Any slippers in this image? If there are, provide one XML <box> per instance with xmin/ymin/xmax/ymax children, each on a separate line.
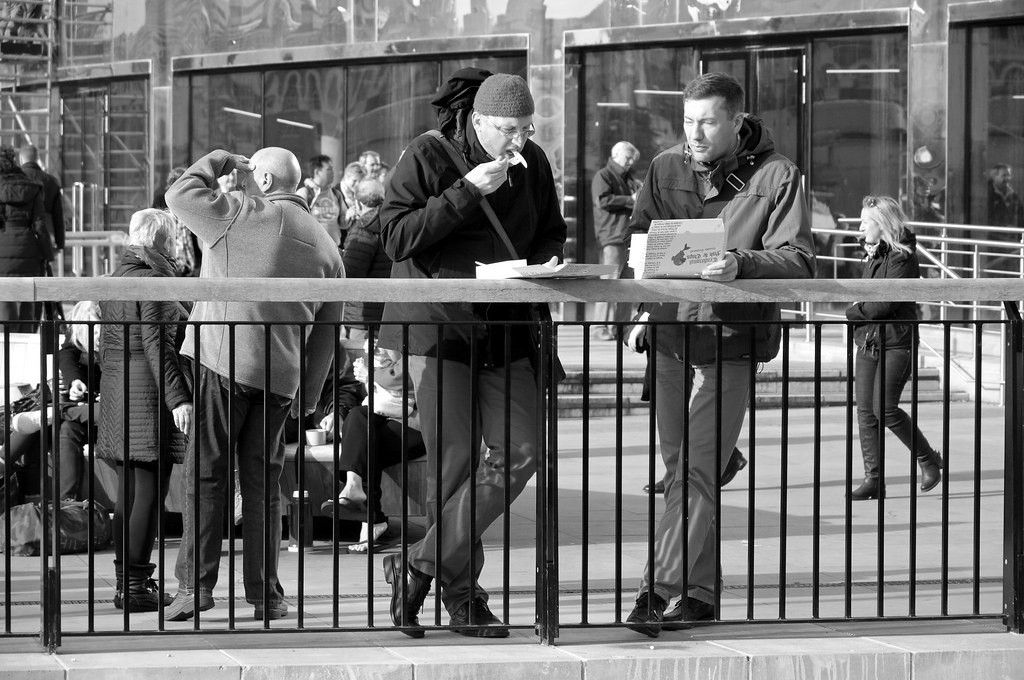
<box><xmin>347</xmin><ymin>532</ymin><xmax>400</xmax><ymax>555</ymax></box>
<box><xmin>320</xmin><ymin>497</ymin><xmax>366</xmax><ymax>521</ymax></box>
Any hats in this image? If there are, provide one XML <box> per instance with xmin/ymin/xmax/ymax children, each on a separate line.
<box><xmin>474</xmin><ymin>73</ymin><xmax>535</xmax><ymax>118</ymax></box>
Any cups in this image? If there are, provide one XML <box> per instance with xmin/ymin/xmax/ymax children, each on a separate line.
<box><xmin>306</xmin><ymin>428</ymin><xmax>326</xmax><ymax>446</ymax></box>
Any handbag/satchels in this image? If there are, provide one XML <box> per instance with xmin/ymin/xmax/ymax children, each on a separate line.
<box><xmin>0</xmin><ymin>498</ymin><xmax>114</xmax><ymax>559</ymax></box>
<box><xmin>44</xmin><ymin>263</ymin><xmax>68</xmax><ymax>335</ymax></box>
<box><xmin>533</xmin><ymin>318</ymin><xmax>566</xmax><ymax>387</ymax></box>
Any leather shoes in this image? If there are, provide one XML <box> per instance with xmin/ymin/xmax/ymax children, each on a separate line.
<box><xmin>450</xmin><ymin>598</ymin><xmax>510</xmax><ymax>637</ymax></box>
<box><xmin>384</xmin><ymin>553</ymin><xmax>430</xmax><ymax>638</ymax></box>
<box><xmin>721</xmin><ymin>448</ymin><xmax>747</xmax><ymax>485</ymax></box>
<box><xmin>644</xmin><ymin>479</ymin><xmax>665</xmax><ymax>494</ymax></box>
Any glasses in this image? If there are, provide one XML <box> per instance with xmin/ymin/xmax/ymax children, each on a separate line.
<box><xmin>484</xmin><ymin>115</ymin><xmax>536</xmax><ymax>141</ymax></box>
<box><xmin>867</xmin><ymin>199</ymin><xmax>879</xmax><ymax>209</ymax></box>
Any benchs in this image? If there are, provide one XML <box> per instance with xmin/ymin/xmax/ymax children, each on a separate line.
<box><xmin>76</xmin><ymin>441</ymin><xmax>431</xmax><ymax>519</ymax></box>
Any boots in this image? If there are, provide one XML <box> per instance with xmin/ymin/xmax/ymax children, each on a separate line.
<box><xmin>122</xmin><ymin>565</ymin><xmax>176</xmax><ymax>612</ymax></box>
<box><xmin>114</xmin><ymin>558</ymin><xmax>123</xmax><ymax>609</ymax></box>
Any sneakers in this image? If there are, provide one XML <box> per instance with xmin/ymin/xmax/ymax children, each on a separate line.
<box><xmin>625</xmin><ymin>591</ymin><xmax>668</xmax><ymax>638</ymax></box>
<box><xmin>254</xmin><ymin>601</ymin><xmax>288</xmax><ymax>620</ymax></box>
<box><xmin>164</xmin><ymin>586</ymin><xmax>215</xmax><ymax>620</ymax></box>
<box><xmin>661</xmin><ymin>597</ymin><xmax>716</xmax><ymax>630</ymax></box>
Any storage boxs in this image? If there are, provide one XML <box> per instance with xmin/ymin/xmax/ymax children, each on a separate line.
<box><xmin>468</xmin><ymin>258</ymin><xmax>616</xmax><ymax>277</ymax></box>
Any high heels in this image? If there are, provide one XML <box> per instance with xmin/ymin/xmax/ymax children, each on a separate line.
<box><xmin>918</xmin><ymin>451</ymin><xmax>944</xmax><ymax>492</ymax></box>
<box><xmin>852</xmin><ymin>477</ymin><xmax>887</xmax><ymax>500</ymax></box>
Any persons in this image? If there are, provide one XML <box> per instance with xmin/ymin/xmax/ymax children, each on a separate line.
<box><xmin>96</xmin><ymin>209</ymin><xmax>193</xmax><ymax>612</ymax></box>
<box><xmin>845</xmin><ymin>196</ymin><xmax>942</xmax><ymax>499</ymax></box>
<box><xmin>591</xmin><ymin>141</ymin><xmax>640</xmax><ymax>340</ymax></box>
<box><xmin>0</xmin><ymin>145</ymin><xmax>65</xmax><ymax>334</ymax></box>
<box><xmin>0</xmin><ymin>299</ymin><xmax>426</xmax><ymax>552</ymax></box>
<box><xmin>988</xmin><ymin>163</ymin><xmax>1023</xmax><ymax>315</ymax></box>
<box><xmin>165</xmin><ymin>147</ymin><xmax>347</xmax><ymax>620</ymax></box>
<box><xmin>623</xmin><ymin>73</ymin><xmax>815</xmax><ymax>631</ymax></box>
<box><xmin>154</xmin><ymin>151</ymin><xmax>394</xmax><ymax>341</ymax></box>
<box><xmin>378</xmin><ymin>66</ymin><xmax>567</xmax><ymax>638</ymax></box>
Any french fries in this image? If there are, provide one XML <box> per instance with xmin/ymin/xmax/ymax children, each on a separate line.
<box><xmin>511</xmin><ymin>150</ymin><xmax>527</xmax><ymax>168</ymax></box>
